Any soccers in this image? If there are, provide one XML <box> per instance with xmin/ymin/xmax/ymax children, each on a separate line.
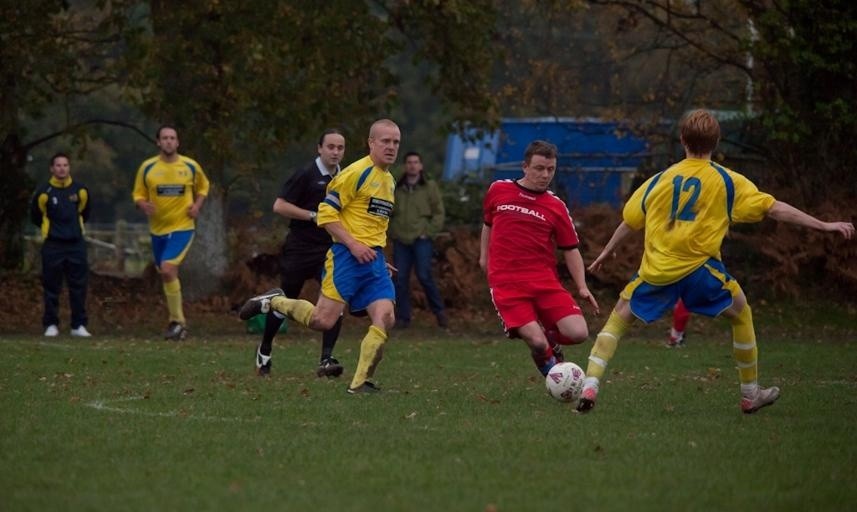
<box><xmin>546</xmin><ymin>362</ymin><xmax>586</xmax><ymax>403</ymax></box>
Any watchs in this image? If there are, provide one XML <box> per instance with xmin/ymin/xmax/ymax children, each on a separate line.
<box><xmin>310</xmin><ymin>211</ymin><xmax>316</xmax><ymax>222</ymax></box>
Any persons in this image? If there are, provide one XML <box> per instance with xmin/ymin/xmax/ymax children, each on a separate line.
<box><xmin>132</xmin><ymin>124</ymin><xmax>211</xmax><ymax>341</ymax></box>
<box><xmin>388</xmin><ymin>151</ymin><xmax>452</xmax><ymax>330</ymax></box>
<box><xmin>254</xmin><ymin>127</ymin><xmax>345</xmax><ymax>377</ymax></box>
<box><xmin>478</xmin><ymin>140</ymin><xmax>601</xmax><ymax>379</ymax></box>
<box><xmin>239</xmin><ymin>119</ymin><xmax>401</xmax><ymax>396</ymax></box>
<box><xmin>29</xmin><ymin>152</ymin><xmax>93</xmax><ymax>338</ymax></box>
<box><xmin>668</xmin><ymin>299</ymin><xmax>689</xmax><ymax>345</ymax></box>
<box><xmin>575</xmin><ymin>107</ymin><xmax>855</xmax><ymax>415</ymax></box>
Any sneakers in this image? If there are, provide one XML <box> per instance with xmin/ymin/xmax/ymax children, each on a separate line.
<box><xmin>346</xmin><ymin>380</ymin><xmax>381</xmax><ymax>395</ymax></box>
<box><xmin>44</xmin><ymin>325</ymin><xmax>59</xmax><ymax>338</ymax></box>
<box><xmin>70</xmin><ymin>325</ymin><xmax>91</xmax><ymax>338</ymax></box>
<box><xmin>317</xmin><ymin>356</ymin><xmax>342</xmax><ymax>378</ymax></box>
<box><xmin>542</xmin><ymin>326</ymin><xmax>564</xmax><ymax>362</ymax></box>
<box><xmin>255</xmin><ymin>342</ymin><xmax>272</xmax><ymax>377</ymax></box>
<box><xmin>164</xmin><ymin>321</ymin><xmax>188</xmax><ymax>341</ymax></box>
<box><xmin>576</xmin><ymin>381</ymin><xmax>599</xmax><ymax>413</ymax></box>
<box><xmin>665</xmin><ymin>332</ymin><xmax>687</xmax><ymax>348</ymax></box>
<box><xmin>239</xmin><ymin>288</ymin><xmax>286</xmax><ymax>321</ymax></box>
<box><xmin>740</xmin><ymin>385</ymin><xmax>780</xmax><ymax>414</ymax></box>
